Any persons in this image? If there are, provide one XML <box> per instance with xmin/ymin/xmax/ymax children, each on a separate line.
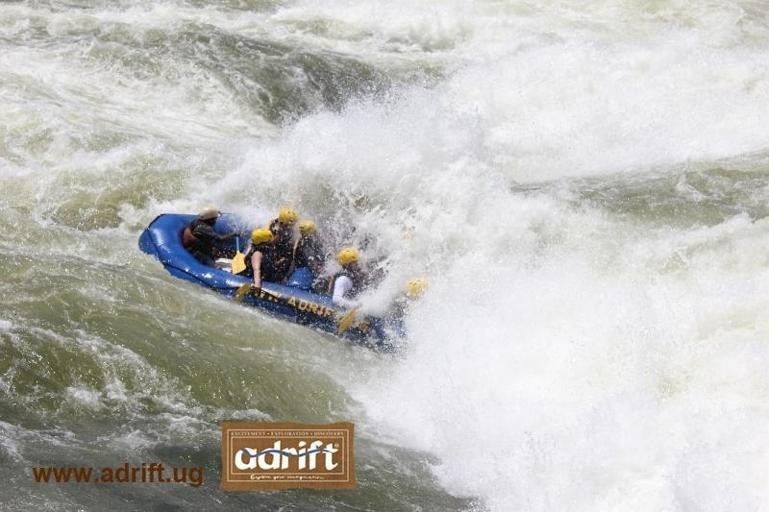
<box><xmin>183</xmin><ymin>203</ymin><xmax>427</xmax><ymax>339</ymax></box>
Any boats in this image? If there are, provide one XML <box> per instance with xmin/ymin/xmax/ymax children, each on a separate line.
<box><xmin>139</xmin><ymin>212</ymin><xmax>407</xmax><ymax>353</ymax></box>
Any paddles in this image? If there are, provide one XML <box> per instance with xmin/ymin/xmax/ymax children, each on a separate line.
<box><xmin>235</xmin><ymin>256</ymin><xmax>288</xmax><ymax>303</ymax></box>
<box><xmin>339</xmin><ymin>303</ymin><xmax>362</xmax><ymax>335</ymax></box>
<box><xmin>231</xmin><ymin>218</ymin><xmax>247</xmax><ymax>275</ymax></box>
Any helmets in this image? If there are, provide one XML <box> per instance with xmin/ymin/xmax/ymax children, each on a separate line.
<box><xmin>337</xmin><ymin>247</ymin><xmax>359</xmax><ymax>266</ymax></box>
<box><xmin>251</xmin><ymin>228</ymin><xmax>274</xmax><ymax>245</ymax></box>
<box><xmin>298</xmin><ymin>220</ymin><xmax>317</xmax><ymax>235</ymax></box>
<box><xmin>199</xmin><ymin>207</ymin><xmax>219</xmax><ymax>220</ymax></box>
<box><xmin>403</xmin><ymin>278</ymin><xmax>430</xmax><ymax>298</ymax></box>
<box><xmin>278</xmin><ymin>207</ymin><xmax>299</xmax><ymax>226</ymax></box>
